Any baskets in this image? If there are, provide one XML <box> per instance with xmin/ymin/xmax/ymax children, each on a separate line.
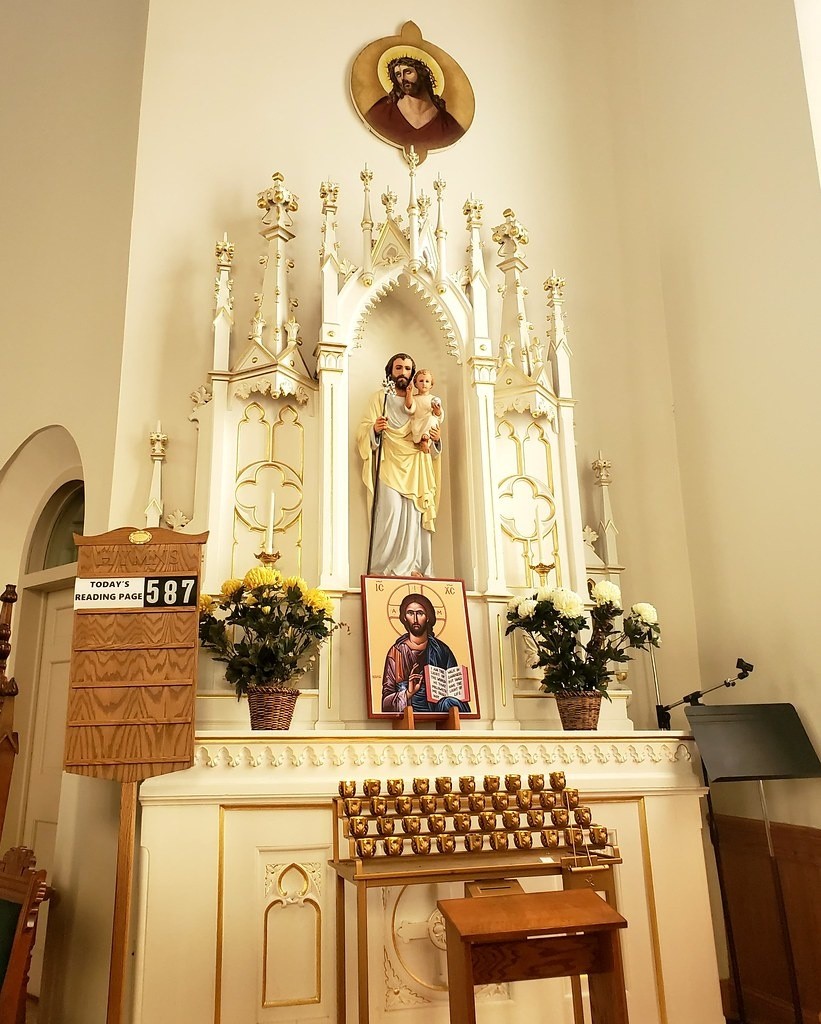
<box><xmin>554</xmin><ymin>691</ymin><xmax>603</xmax><ymax>731</ymax></box>
<box><xmin>247</xmin><ymin>686</ymin><xmax>301</xmax><ymax>730</ymax></box>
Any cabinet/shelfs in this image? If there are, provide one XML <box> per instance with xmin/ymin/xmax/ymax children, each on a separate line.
<box><xmin>328</xmin><ymin>790</ymin><xmax>629</xmax><ymax>1024</ymax></box>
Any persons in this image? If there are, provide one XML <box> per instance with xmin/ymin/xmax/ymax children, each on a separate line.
<box><xmin>404</xmin><ymin>369</ymin><xmax>445</xmax><ymax>455</ymax></box>
<box><xmin>357</xmin><ymin>352</ymin><xmax>442</xmax><ymax>578</ymax></box>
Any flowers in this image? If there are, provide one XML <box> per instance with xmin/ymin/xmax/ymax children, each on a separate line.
<box><xmin>199</xmin><ymin>566</ymin><xmax>350</xmax><ymax>703</ymax></box>
<box><xmin>503</xmin><ymin>579</ymin><xmax>662</xmax><ymax>703</ymax></box>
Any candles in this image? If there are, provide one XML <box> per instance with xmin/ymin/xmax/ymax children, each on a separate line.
<box><xmin>536</xmin><ymin>503</ymin><xmax>545</xmax><ymax>565</ymax></box>
<box><xmin>265</xmin><ymin>491</ymin><xmax>274</xmax><ymax>555</ymax></box>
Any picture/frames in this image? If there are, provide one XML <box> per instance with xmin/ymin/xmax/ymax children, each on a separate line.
<box><xmin>361</xmin><ymin>574</ymin><xmax>481</xmax><ymax>719</ymax></box>
